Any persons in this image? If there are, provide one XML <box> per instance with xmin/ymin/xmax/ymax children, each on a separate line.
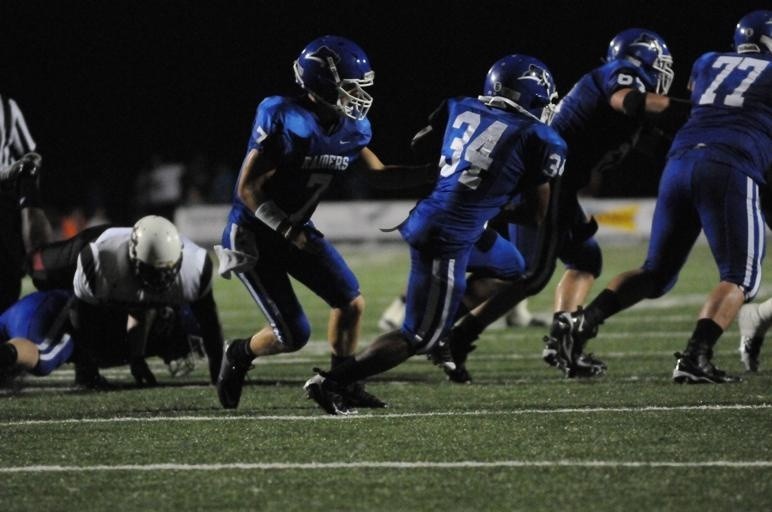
<box><xmin>738</xmin><ymin>293</ymin><xmax>772</xmax><ymax>372</ymax></box>
<box><xmin>550</xmin><ymin>10</ymin><xmax>771</xmax><ymax>386</ymax></box>
<box><xmin>1</xmin><ymin>85</ymin><xmax>42</xmax><ymax>313</ymax></box>
<box><xmin>378</xmin><ymin>195</ymin><xmax>552</xmax><ymax>335</ymax></box>
<box><xmin>215</xmin><ymin>34</ymin><xmax>439</xmax><ymax>410</ymax></box>
<box><xmin>303</xmin><ymin>54</ymin><xmax>569</xmax><ymax>418</ymax></box>
<box><xmin>427</xmin><ymin>27</ymin><xmax>695</xmax><ymax>386</ymax></box>
<box><xmin>2</xmin><ymin>289</ymin><xmax>216</xmax><ymax>376</ymax></box>
<box><xmin>0</xmin><ymin>152</ymin><xmax>224</xmax><ymax>391</ymax></box>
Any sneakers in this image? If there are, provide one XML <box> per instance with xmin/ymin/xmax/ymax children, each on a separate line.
<box><xmin>323</xmin><ymin>356</ymin><xmax>388</xmax><ymax>410</ymax></box>
<box><xmin>302</xmin><ymin>374</ymin><xmax>351</xmax><ymax>416</ymax></box>
<box><xmin>738</xmin><ymin>303</ymin><xmax>768</xmax><ymax>373</ymax></box>
<box><xmin>66</xmin><ymin>371</ymin><xmax>107</xmax><ymax>394</ymax></box>
<box><xmin>216</xmin><ymin>337</ymin><xmax>253</xmax><ymax>409</ymax></box>
<box><xmin>1</xmin><ymin>344</ymin><xmax>20</xmax><ymax>388</ymax></box>
<box><xmin>445</xmin><ymin>340</ymin><xmax>473</xmax><ymax>383</ymax></box>
<box><xmin>129</xmin><ymin>356</ymin><xmax>156</xmax><ymax>387</ymax></box>
<box><xmin>377</xmin><ymin>297</ymin><xmax>407</xmax><ymax>334</ymax></box>
<box><xmin>673</xmin><ymin>356</ymin><xmax>744</xmax><ymax>385</ymax></box>
<box><xmin>508</xmin><ymin>313</ymin><xmax>549</xmax><ymax>330</ymax></box>
<box><xmin>551</xmin><ymin>309</ymin><xmax>596</xmax><ymax>367</ymax></box>
<box><xmin>428</xmin><ymin>332</ymin><xmax>456</xmax><ymax>371</ymax></box>
<box><xmin>540</xmin><ymin>326</ymin><xmax>608</xmax><ymax>378</ymax></box>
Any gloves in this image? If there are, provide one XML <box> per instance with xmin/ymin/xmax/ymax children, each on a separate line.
<box><xmin>258</xmin><ymin>203</ymin><xmax>323</xmax><ymax>249</ymax></box>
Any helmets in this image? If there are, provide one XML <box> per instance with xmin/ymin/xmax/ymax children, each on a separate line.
<box><xmin>477</xmin><ymin>52</ymin><xmax>558</xmax><ymax>126</ymax></box>
<box><xmin>293</xmin><ymin>33</ymin><xmax>377</xmax><ymax>121</ymax></box>
<box><xmin>733</xmin><ymin>10</ymin><xmax>772</xmax><ymax>60</ymax></box>
<box><xmin>605</xmin><ymin>28</ymin><xmax>674</xmax><ymax>97</ymax></box>
<box><xmin>130</xmin><ymin>215</ymin><xmax>185</xmax><ymax>291</ymax></box>
